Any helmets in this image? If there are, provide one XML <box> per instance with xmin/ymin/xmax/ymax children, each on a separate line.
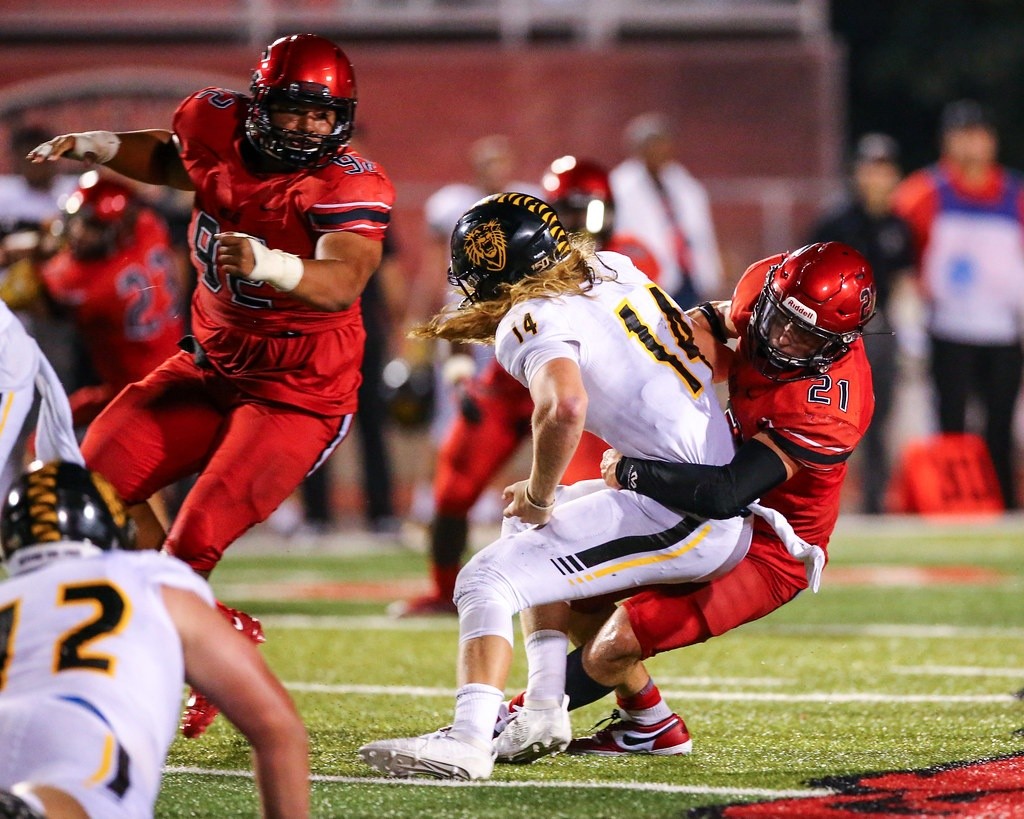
<box><xmin>451</xmin><ymin>191</ymin><xmax>571</xmax><ymax>300</ymax></box>
<box><xmin>543</xmin><ymin>154</ymin><xmax>613</xmax><ymax>209</ymax></box>
<box><xmin>66</xmin><ymin>178</ymin><xmax>137</xmax><ymax>261</ymax></box>
<box><xmin>1</xmin><ymin>458</ymin><xmax>127</xmax><ymax>560</ymax></box>
<box><xmin>245</xmin><ymin>31</ymin><xmax>357</xmax><ymax>171</ymax></box>
<box><xmin>747</xmin><ymin>241</ymin><xmax>878</xmax><ymax>384</ymax></box>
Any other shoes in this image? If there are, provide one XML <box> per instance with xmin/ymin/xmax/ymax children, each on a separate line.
<box><xmin>388</xmin><ymin>594</ymin><xmax>457</xmax><ymax>619</ymax></box>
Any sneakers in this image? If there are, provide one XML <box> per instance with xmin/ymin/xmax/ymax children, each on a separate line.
<box><xmin>434</xmin><ymin>698</ymin><xmax>514</xmax><ymax>740</ymax></box>
<box><xmin>492</xmin><ymin>695</ymin><xmax>571</xmax><ymax>764</ymax></box>
<box><xmin>564</xmin><ymin>708</ymin><xmax>693</xmax><ymax>756</ymax></box>
<box><xmin>357</xmin><ymin>726</ymin><xmax>499</xmax><ymax>779</ymax></box>
<box><xmin>180</xmin><ymin>608</ymin><xmax>266</xmax><ymax>739</ymax></box>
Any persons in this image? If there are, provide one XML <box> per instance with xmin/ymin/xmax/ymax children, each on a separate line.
<box><xmin>358</xmin><ymin>190</ymin><xmax>759</xmax><ymax>781</ymax></box>
<box><xmin>0</xmin><ymin>30</ymin><xmax>397</xmax><ymax>740</ymax></box>
<box><xmin>398</xmin><ymin>110</ymin><xmax>727</xmax><ymax>554</ymax></box>
<box><xmin>387</xmin><ymin>152</ymin><xmax>663</xmax><ymax>618</ymax></box>
<box><xmin>439</xmin><ymin>241</ymin><xmax>874</xmax><ymax>741</ymax></box>
<box><xmin>798</xmin><ymin>98</ymin><xmax>1024</xmax><ymax>514</ymax></box>
<box><xmin>0</xmin><ymin>298</ymin><xmax>89</xmax><ymax>567</ymax></box>
<box><xmin>0</xmin><ymin>463</ymin><xmax>310</xmax><ymax>819</ymax></box>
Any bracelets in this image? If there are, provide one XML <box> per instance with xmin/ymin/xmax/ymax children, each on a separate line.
<box><xmin>524</xmin><ymin>484</ymin><xmax>557</xmax><ymax>512</ymax></box>
